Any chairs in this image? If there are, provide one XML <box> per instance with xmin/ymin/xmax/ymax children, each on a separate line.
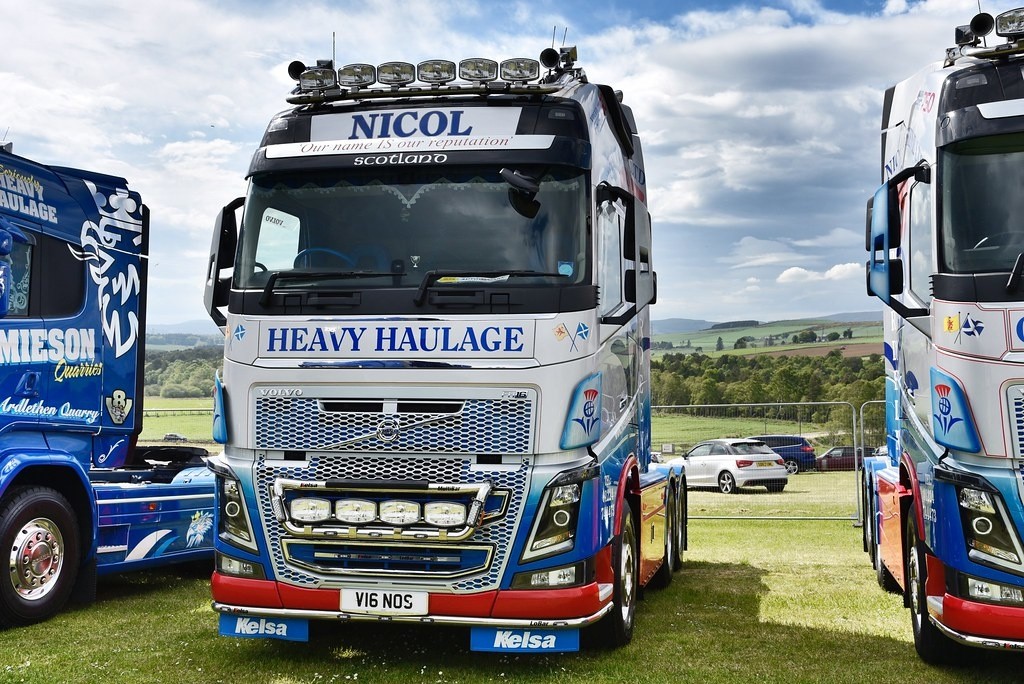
<box><xmin>338</xmin><ymin>240</ymin><xmax>396</xmax><ymax>275</ymax></box>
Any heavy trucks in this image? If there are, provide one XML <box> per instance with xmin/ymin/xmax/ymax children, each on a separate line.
<box><xmin>198</xmin><ymin>36</ymin><xmax>692</xmax><ymax>661</ymax></box>
<box><xmin>852</xmin><ymin>1</ymin><xmax>1024</xmax><ymax>670</ymax></box>
<box><xmin>0</xmin><ymin>143</ymin><xmax>220</xmax><ymax>626</ymax></box>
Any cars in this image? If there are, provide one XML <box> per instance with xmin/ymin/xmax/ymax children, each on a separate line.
<box><xmin>665</xmin><ymin>436</ymin><xmax>790</xmax><ymax>494</ymax></box>
<box><xmin>814</xmin><ymin>444</ymin><xmax>868</xmax><ymax>472</ymax></box>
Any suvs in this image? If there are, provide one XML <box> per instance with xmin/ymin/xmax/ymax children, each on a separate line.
<box><xmin>736</xmin><ymin>433</ymin><xmax>818</xmax><ymax>476</ymax></box>
